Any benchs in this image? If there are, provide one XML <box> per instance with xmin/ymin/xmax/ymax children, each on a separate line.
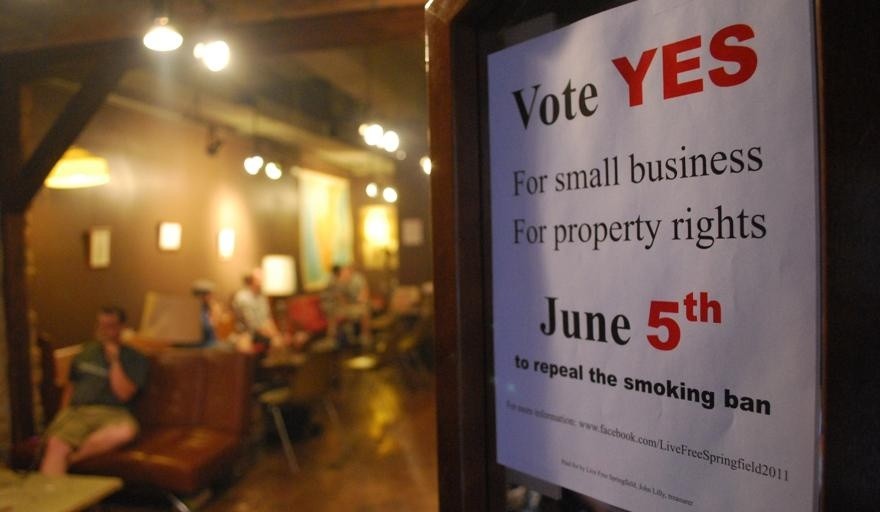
<box><xmin>7</xmin><ymin>331</ymin><xmax>257</xmax><ymax>500</ymax></box>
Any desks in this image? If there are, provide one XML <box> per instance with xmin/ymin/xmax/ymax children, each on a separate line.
<box><xmin>0</xmin><ymin>468</ymin><xmax>125</xmax><ymax>511</ymax></box>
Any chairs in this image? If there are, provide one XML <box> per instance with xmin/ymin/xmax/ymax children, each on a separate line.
<box><xmin>256</xmin><ymin>332</ymin><xmax>391</xmax><ymax>475</ymax></box>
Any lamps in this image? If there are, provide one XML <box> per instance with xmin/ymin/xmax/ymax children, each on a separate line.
<box><xmin>42</xmin><ymin>142</ymin><xmax>112</xmax><ymax>191</ymax></box>
<box><xmin>142</xmin><ymin>0</ymin><xmax>184</xmax><ymax>52</ymax></box>
